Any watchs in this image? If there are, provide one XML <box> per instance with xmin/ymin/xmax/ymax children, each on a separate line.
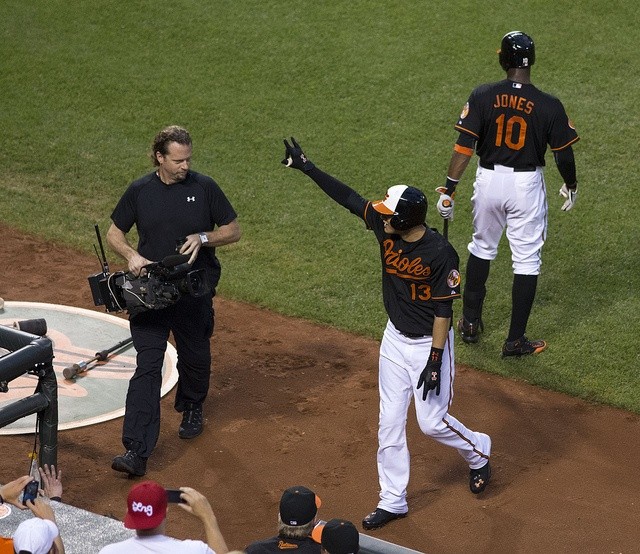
<box><xmin>199</xmin><ymin>232</ymin><xmax>209</xmax><ymax>245</ymax></box>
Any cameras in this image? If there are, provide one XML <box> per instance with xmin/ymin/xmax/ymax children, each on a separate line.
<box><xmin>88</xmin><ymin>224</ymin><xmax>211</xmax><ymax>314</ymax></box>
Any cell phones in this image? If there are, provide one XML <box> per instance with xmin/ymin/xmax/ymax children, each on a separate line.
<box><xmin>22</xmin><ymin>480</ymin><xmax>39</xmax><ymax>507</ymax></box>
<box><xmin>164</xmin><ymin>488</ymin><xmax>188</xmax><ymax>503</ymax></box>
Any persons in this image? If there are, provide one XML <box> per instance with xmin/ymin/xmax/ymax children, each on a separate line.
<box><xmin>98</xmin><ymin>479</ymin><xmax>228</xmax><ymax>554</ymax></box>
<box><xmin>281</xmin><ymin>136</ymin><xmax>491</xmax><ymax>529</ymax></box>
<box><xmin>0</xmin><ymin>474</ymin><xmax>65</xmax><ymax>554</ymax></box>
<box><xmin>320</xmin><ymin>519</ymin><xmax>361</xmax><ymax>553</ymax></box>
<box><xmin>36</xmin><ymin>462</ymin><xmax>63</xmax><ymax>502</ymax></box>
<box><xmin>105</xmin><ymin>124</ymin><xmax>243</xmax><ymax>475</ymax></box>
<box><xmin>243</xmin><ymin>484</ymin><xmax>325</xmax><ymax>554</ymax></box>
<box><xmin>436</xmin><ymin>29</ymin><xmax>581</xmax><ymax>358</ymax></box>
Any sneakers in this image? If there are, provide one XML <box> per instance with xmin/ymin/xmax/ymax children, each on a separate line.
<box><xmin>457</xmin><ymin>319</ymin><xmax>481</xmax><ymax>343</ymax></box>
<box><xmin>362</xmin><ymin>508</ymin><xmax>409</xmax><ymax>530</ymax></box>
<box><xmin>111</xmin><ymin>449</ymin><xmax>147</xmax><ymax>477</ymax></box>
<box><xmin>469</xmin><ymin>460</ymin><xmax>491</xmax><ymax>494</ymax></box>
<box><xmin>179</xmin><ymin>404</ymin><xmax>203</xmax><ymax>439</ymax></box>
<box><xmin>502</xmin><ymin>336</ymin><xmax>547</xmax><ymax>356</ymax></box>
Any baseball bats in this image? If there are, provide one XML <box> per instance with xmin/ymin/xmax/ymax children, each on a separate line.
<box><xmin>442</xmin><ymin>199</ymin><xmax>451</xmax><ymax>238</ymax></box>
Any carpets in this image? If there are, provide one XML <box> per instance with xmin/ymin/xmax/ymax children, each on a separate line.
<box><xmin>0</xmin><ymin>487</ymin><xmax>137</xmax><ymax>554</ymax></box>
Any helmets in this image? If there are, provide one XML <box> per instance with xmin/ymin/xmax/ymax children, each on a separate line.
<box><xmin>498</xmin><ymin>30</ymin><xmax>534</xmax><ymax>66</ymax></box>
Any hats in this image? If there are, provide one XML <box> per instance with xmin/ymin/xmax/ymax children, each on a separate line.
<box><xmin>372</xmin><ymin>184</ymin><xmax>428</xmax><ymax>229</ymax></box>
<box><xmin>124</xmin><ymin>480</ymin><xmax>167</xmax><ymax>531</ymax></box>
<box><xmin>312</xmin><ymin>518</ymin><xmax>359</xmax><ymax>554</ymax></box>
<box><xmin>280</xmin><ymin>486</ymin><xmax>322</xmax><ymax>527</ymax></box>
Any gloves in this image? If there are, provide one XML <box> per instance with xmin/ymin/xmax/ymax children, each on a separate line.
<box><xmin>434</xmin><ymin>186</ymin><xmax>455</xmax><ymax>221</ymax></box>
<box><xmin>281</xmin><ymin>138</ymin><xmax>314</xmax><ymax>175</ymax></box>
<box><xmin>559</xmin><ymin>183</ymin><xmax>578</xmax><ymax>212</ymax></box>
<box><xmin>417</xmin><ymin>346</ymin><xmax>445</xmax><ymax>401</ymax></box>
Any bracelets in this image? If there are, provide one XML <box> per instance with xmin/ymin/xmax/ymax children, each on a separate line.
<box><xmin>445</xmin><ymin>176</ymin><xmax>459</xmax><ymax>196</ymax></box>
<box><xmin>427</xmin><ymin>346</ymin><xmax>444</xmax><ymax>366</ymax></box>
